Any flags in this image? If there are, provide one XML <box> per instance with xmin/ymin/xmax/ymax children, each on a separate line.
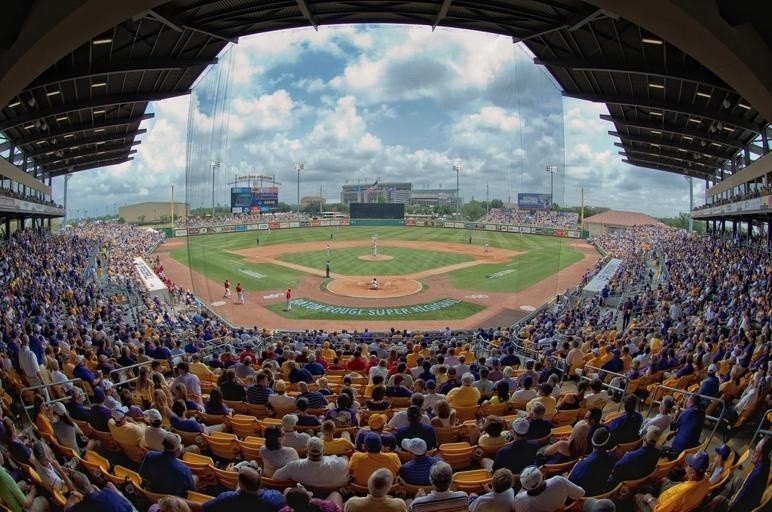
<box><xmin>367</xmin><ymin>182</ymin><xmax>377</xmax><ymax>192</ymax></box>
<box><xmin>388</xmin><ymin>186</ymin><xmax>394</xmax><ymax>191</ymax></box>
<box><xmin>351</xmin><ymin>185</ymin><xmax>359</xmax><ymax>192</ymax></box>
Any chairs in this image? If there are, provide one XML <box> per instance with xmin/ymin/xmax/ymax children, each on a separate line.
<box><xmin>0</xmin><ymin>221</ymin><xmax>772</xmax><ymax>511</ymax></box>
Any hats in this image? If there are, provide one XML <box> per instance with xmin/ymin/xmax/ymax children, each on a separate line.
<box><xmin>307</xmin><ymin>437</ymin><xmax>324</xmax><ymax>455</ymax></box>
<box><xmin>686</xmin><ymin>444</ymin><xmax>732</xmax><ymax>467</ymax></box>
<box><xmin>647</xmin><ymin>425</ymin><xmax>660</xmax><ymax>445</ymax></box>
<box><xmin>520</xmin><ymin>465</ymin><xmax>542</xmax><ymax>490</ymax></box>
<box><xmin>365</xmin><ymin>414</ymin><xmax>388</xmax><ymax>450</ymax></box>
<box><xmin>282</xmin><ymin>414</ymin><xmax>299</xmax><ymax>428</ymax></box>
<box><xmin>708</xmin><ymin>364</ymin><xmax>719</xmax><ymax>373</ymax></box>
<box><xmin>101</xmin><ymin>379</ymin><xmax>114</xmax><ymax>391</ymax></box>
<box><xmin>401</xmin><ymin>438</ymin><xmax>427</xmax><ymax>455</ymax></box>
<box><xmin>592</xmin><ymin>427</ymin><xmax>616</xmax><ymax>450</ymax></box>
<box><xmin>110</xmin><ymin>405</ymin><xmax>162</xmax><ymax>425</ymax></box>
<box><xmin>53</xmin><ymin>402</ymin><xmax>66</xmax><ymax>416</ymax></box>
<box><xmin>163</xmin><ymin>434</ymin><xmax>181</xmax><ymax>451</ymax></box>
<box><xmin>513</xmin><ymin>418</ymin><xmax>529</xmax><ymax>434</ymax></box>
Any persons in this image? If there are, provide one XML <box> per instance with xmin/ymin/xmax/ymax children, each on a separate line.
<box><xmin>0</xmin><ymin>208</ymin><xmax>771</xmax><ymax>510</ymax></box>
